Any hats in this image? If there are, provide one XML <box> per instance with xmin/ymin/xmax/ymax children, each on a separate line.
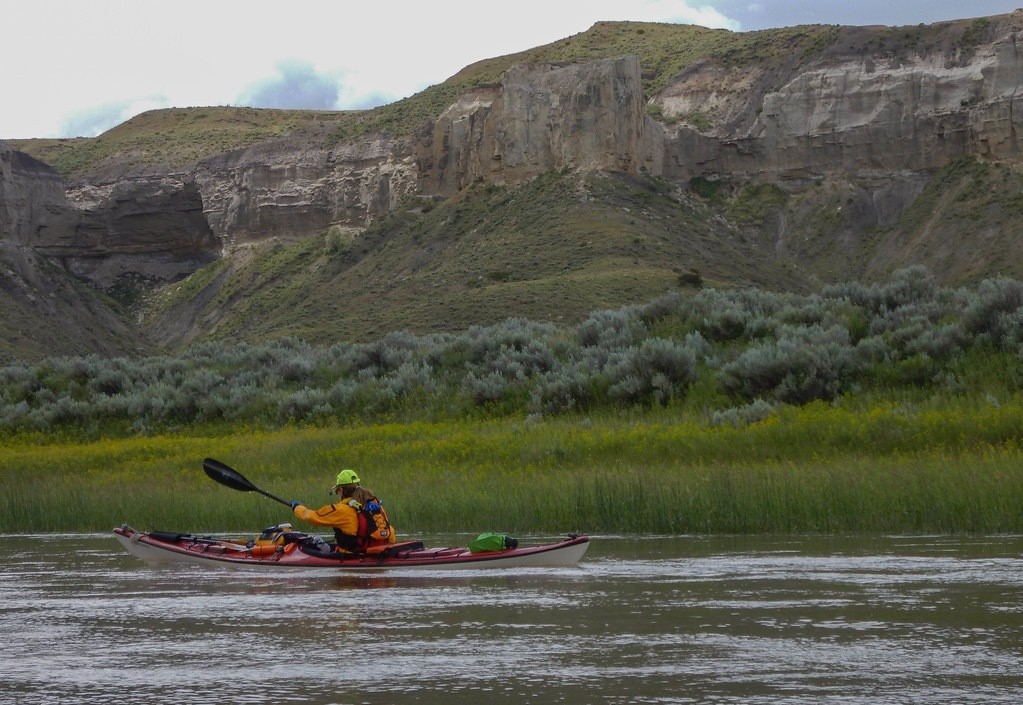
<box><xmin>331</xmin><ymin>470</ymin><xmax>360</xmax><ymax>490</ymax></box>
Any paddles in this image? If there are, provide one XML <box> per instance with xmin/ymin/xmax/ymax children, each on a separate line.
<box><xmin>202</xmin><ymin>456</ymin><xmax>292</xmax><ymax>508</ymax></box>
<box><xmin>140</xmin><ymin>530</ymin><xmax>249</xmax><ymax>551</ymax></box>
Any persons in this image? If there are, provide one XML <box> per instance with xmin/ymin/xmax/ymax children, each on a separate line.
<box><xmin>291</xmin><ymin>469</ymin><xmax>396</xmax><ymax>557</ymax></box>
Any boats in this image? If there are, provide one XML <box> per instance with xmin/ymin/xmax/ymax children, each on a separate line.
<box><xmin>112</xmin><ymin>523</ymin><xmax>591</xmax><ymax>571</ymax></box>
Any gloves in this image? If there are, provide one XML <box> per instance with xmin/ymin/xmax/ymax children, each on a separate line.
<box><xmin>291</xmin><ymin>500</ymin><xmax>300</xmax><ymax>509</ymax></box>
